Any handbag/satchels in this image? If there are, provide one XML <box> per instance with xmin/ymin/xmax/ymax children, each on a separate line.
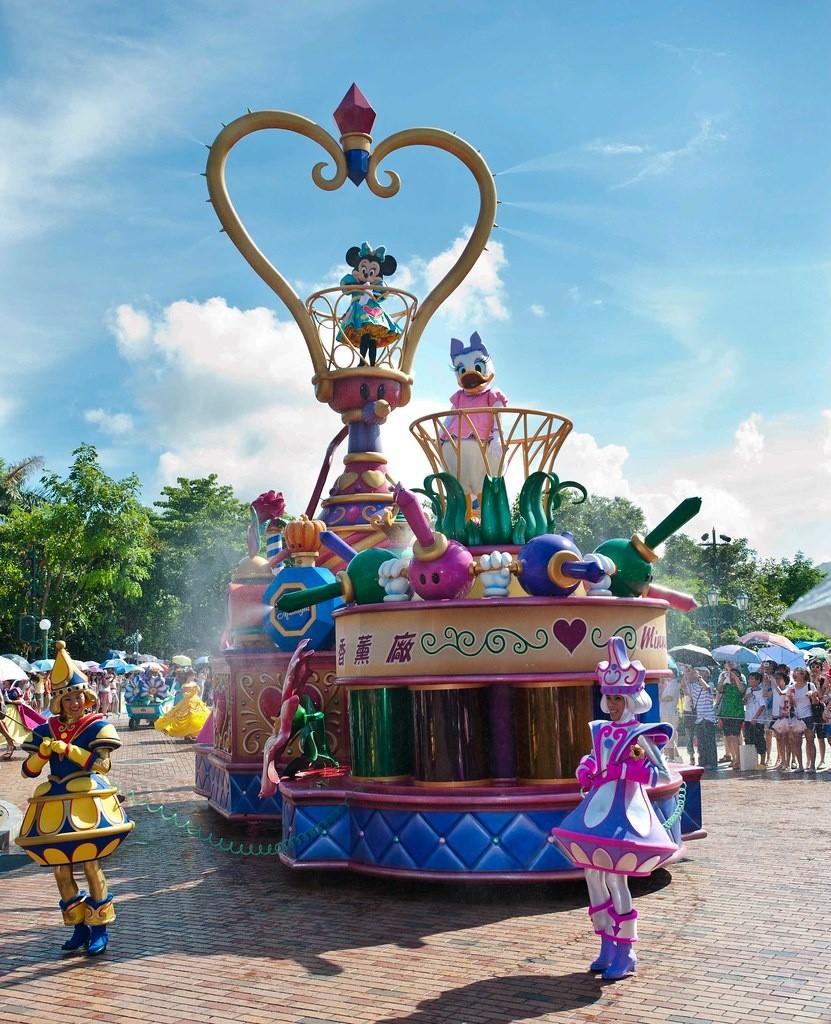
<box><xmin>690</xmin><ymin>709</ymin><xmax>698</xmax><ymax>721</ymax></box>
<box><xmin>812</xmin><ymin>702</ymin><xmax>825</xmax><ymax>723</ymax></box>
<box><xmin>715</xmin><ymin>702</ymin><xmax>723</xmax><ymax>716</ymax></box>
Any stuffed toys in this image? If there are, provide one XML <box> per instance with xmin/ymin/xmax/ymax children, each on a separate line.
<box><xmin>335</xmin><ymin>242</ymin><xmax>397</xmax><ymax>369</ymax></box>
<box><xmin>434</xmin><ymin>331</ymin><xmax>508</xmax><ymax>510</ymax></box>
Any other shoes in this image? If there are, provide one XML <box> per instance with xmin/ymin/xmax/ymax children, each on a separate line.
<box><xmin>733</xmin><ymin>762</ymin><xmax>741</xmax><ymax>770</ymax></box>
<box><xmin>817</xmin><ymin>762</ymin><xmax>828</xmax><ymax>769</ymax></box>
<box><xmin>828</xmin><ymin>768</ymin><xmax>831</xmax><ymax>774</ymax></box>
<box><xmin>704</xmin><ymin>764</ymin><xmax>719</xmax><ymax>771</ymax></box>
<box><xmin>760</xmin><ymin>761</ymin><xmax>817</xmax><ymax>773</ymax></box>
<box><xmin>754</xmin><ymin>763</ymin><xmax>768</xmax><ymax>772</ymax></box>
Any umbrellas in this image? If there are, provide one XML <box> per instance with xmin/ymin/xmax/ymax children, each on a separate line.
<box><xmin>772</xmin><ymin>707</ymin><xmax>807</xmax><ymax>734</ymax></box>
<box><xmin>668</xmin><ymin>630</ymin><xmax>831</xmax><ymax>671</ymax></box>
<box><xmin>0</xmin><ymin>650</ymin><xmax>210</xmax><ymax>681</ymax></box>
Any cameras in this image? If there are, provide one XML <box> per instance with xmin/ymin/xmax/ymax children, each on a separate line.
<box><xmin>724</xmin><ymin>673</ymin><xmax>731</xmax><ymax>677</ymax></box>
<box><xmin>764</xmin><ymin>662</ymin><xmax>769</xmax><ymax>666</ymax></box>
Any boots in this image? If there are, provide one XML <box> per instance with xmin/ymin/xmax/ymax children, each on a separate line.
<box><xmin>589</xmin><ymin>897</ymin><xmax>620</xmax><ymax>972</ymax></box>
<box><xmin>601</xmin><ymin>905</ymin><xmax>641</xmax><ymax>981</ymax></box>
<box><xmin>84</xmin><ymin>892</ymin><xmax>116</xmax><ymax>955</ymax></box>
<box><xmin>57</xmin><ymin>890</ymin><xmax>98</xmax><ymax>952</ymax></box>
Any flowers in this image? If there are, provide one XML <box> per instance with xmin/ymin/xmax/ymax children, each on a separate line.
<box><xmin>124</xmin><ymin>675</ymin><xmax>170</xmax><ymax>705</ymax></box>
<box><xmin>258</xmin><ymin>637</ymin><xmax>339</xmax><ymax>801</ymax></box>
<box><xmin>250</xmin><ymin>490</ymin><xmax>286</xmax><ymax>526</ymax></box>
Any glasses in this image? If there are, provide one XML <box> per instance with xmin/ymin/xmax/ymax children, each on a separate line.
<box><xmin>812</xmin><ymin>665</ymin><xmax>821</xmax><ymax>669</ymax></box>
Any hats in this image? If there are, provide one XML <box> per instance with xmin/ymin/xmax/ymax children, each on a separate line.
<box><xmin>598</xmin><ymin>635</ymin><xmax>647</xmax><ymax>693</ymax></box>
<box><xmin>695</xmin><ymin>666</ymin><xmax>711</xmax><ymax>676</ymax></box>
<box><xmin>46</xmin><ymin>641</ymin><xmax>98</xmax><ymax>713</ymax></box>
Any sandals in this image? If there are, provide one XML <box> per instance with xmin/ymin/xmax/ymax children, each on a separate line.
<box><xmin>718</xmin><ymin>755</ymin><xmax>732</xmax><ymax>764</ymax></box>
<box><xmin>728</xmin><ymin>760</ymin><xmax>736</xmax><ymax>767</ymax></box>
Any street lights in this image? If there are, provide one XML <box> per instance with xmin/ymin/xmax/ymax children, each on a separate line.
<box><xmin>39</xmin><ymin>618</ymin><xmax>52</xmax><ymax>661</ymax></box>
<box><xmin>736</xmin><ymin>589</ymin><xmax>749</xmax><ymax>638</ymax></box>
<box><xmin>134</xmin><ymin>633</ymin><xmax>143</xmax><ymax>665</ymax></box>
<box><xmin>706</xmin><ymin>585</ymin><xmax>719</xmax><ymax>650</ymax></box>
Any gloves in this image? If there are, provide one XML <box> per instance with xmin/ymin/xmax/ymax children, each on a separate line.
<box><xmin>606</xmin><ymin>760</ymin><xmax>651</xmax><ymax>784</ymax></box>
<box><xmin>49</xmin><ymin>739</ymin><xmax>99</xmax><ymax>772</ymax></box>
<box><xmin>577</xmin><ymin>766</ymin><xmax>595</xmax><ymax>788</ymax></box>
<box><xmin>22</xmin><ymin>738</ymin><xmax>54</xmax><ymax>779</ymax></box>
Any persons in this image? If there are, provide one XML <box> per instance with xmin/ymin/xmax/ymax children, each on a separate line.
<box><xmin>0</xmin><ymin>664</ymin><xmax>213</xmax><ymax>758</ymax></box>
<box><xmin>15</xmin><ymin>643</ymin><xmax>123</xmax><ymax>955</ymax></box>
<box><xmin>551</xmin><ymin>636</ymin><xmax>678</xmax><ymax>983</ymax></box>
<box><xmin>659</xmin><ymin>657</ymin><xmax>831</xmax><ymax>773</ymax></box>
